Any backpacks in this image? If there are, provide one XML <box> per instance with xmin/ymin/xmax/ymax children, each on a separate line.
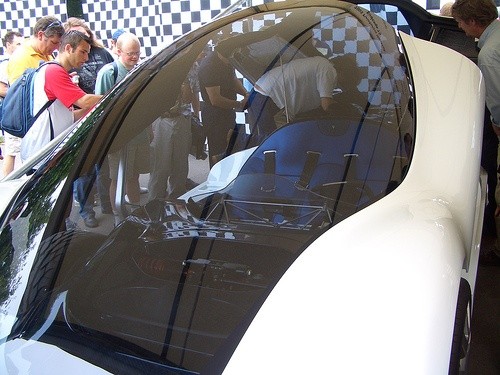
<box><xmin>0</xmin><ymin>59</ymin><xmax>67</xmax><ymax>137</ymax></box>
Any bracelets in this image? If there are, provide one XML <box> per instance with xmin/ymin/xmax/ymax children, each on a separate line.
<box><xmin>243</xmin><ymin>92</ymin><xmax>248</xmax><ymax>96</ymax></box>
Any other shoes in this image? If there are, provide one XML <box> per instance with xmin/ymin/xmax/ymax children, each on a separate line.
<box><xmin>480</xmin><ymin>249</ymin><xmax>500</xmax><ymax>267</ymax></box>
<box><xmin>137</xmin><ymin>184</ymin><xmax>148</xmax><ymax>193</ymax></box>
<box><xmin>74</xmin><ymin>198</ymin><xmax>80</xmax><ymax>206</ymax></box>
<box><xmin>101</xmin><ymin>206</ymin><xmax>114</xmax><ymax>214</ymax></box>
<box><xmin>83</xmin><ymin>213</ymin><xmax>100</xmax><ymax>229</ymax></box>
<box><xmin>186</xmin><ymin>178</ymin><xmax>198</xmax><ymax>190</ymax></box>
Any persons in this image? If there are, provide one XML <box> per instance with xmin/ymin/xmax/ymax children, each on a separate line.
<box><xmin>0</xmin><ymin>17</ymin><xmax>210</xmax><ymax>230</ymax></box>
<box><xmin>198</xmin><ymin>33</ymin><xmax>362</xmax><ymax>170</ymax></box>
<box><xmin>439</xmin><ymin>0</ymin><xmax>500</xmax><ymax>217</ymax></box>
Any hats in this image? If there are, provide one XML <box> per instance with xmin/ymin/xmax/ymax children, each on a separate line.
<box><xmin>112</xmin><ymin>29</ymin><xmax>125</xmax><ymax>40</ymax></box>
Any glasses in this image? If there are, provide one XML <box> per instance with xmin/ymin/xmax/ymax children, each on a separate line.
<box><xmin>119</xmin><ymin>48</ymin><xmax>140</xmax><ymax>55</ymax></box>
<box><xmin>45</xmin><ymin>20</ymin><xmax>62</xmax><ymax>32</ymax></box>
